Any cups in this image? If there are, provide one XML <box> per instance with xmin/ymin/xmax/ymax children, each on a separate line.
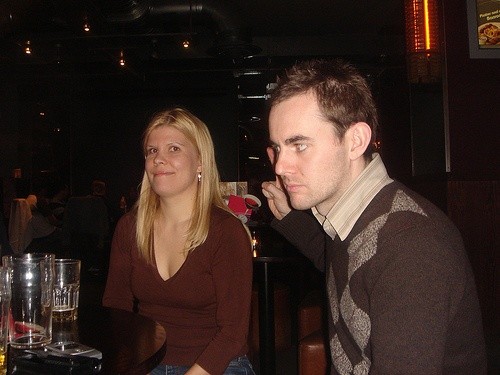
<box><xmin>2</xmin><ymin>252</ymin><xmax>55</xmax><ymax>348</ymax></box>
<box><xmin>0</xmin><ymin>267</ymin><xmax>14</xmax><ymax>375</ymax></box>
<box><xmin>40</xmin><ymin>259</ymin><xmax>81</xmax><ymax>350</ymax></box>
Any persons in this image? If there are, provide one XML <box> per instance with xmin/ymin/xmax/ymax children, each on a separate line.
<box><xmin>102</xmin><ymin>107</ymin><xmax>257</xmax><ymax>375</ymax></box>
<box><xmin>261</xmin><ymin>53</ymin><xmax>488</xmax><ymax>375</ymax></box>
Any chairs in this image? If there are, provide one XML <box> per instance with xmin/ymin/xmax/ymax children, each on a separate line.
<box><xmin>253</xmin><ymin>257</ymin><xmax>279</xmax><ymax>375</ymax></box>
<box><xmin>9</xmin><ymin>198</ymin><xmax>33</xmax><ymax>257</ymax></box>
<box><xmin>62</xmin><ymin>196</ymin><xmax>112</xmax><ymax>283</ymax></box>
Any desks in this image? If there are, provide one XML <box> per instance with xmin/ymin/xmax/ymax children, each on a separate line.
<box><xmin>0</xmin><ymin>300</ymin><xmax>167</xmax><ymax>375</ymax></box>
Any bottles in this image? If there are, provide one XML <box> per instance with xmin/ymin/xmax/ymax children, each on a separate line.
<box><xmin>252</xmin><ymin>232</ymin><xmax>257</xmax><ymax>259</ymax></box>
